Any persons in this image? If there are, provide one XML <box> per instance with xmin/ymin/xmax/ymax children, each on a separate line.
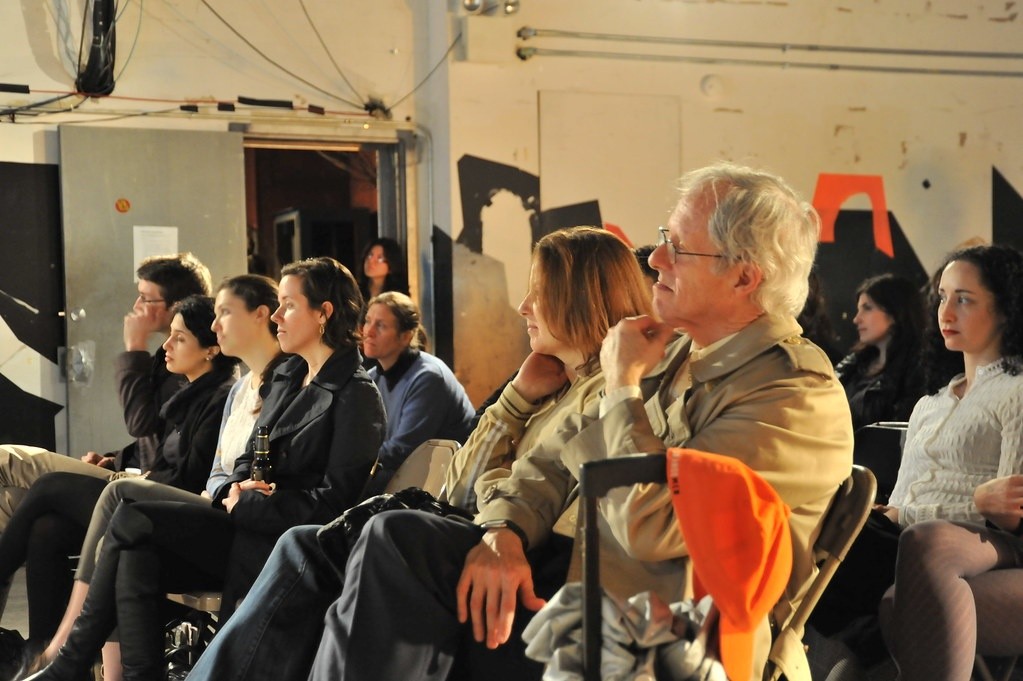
<box><xmin>23</xmin><ymin>259</ymin><xmax>386</xmax><ymax>681</ymax></box>
<box><xmin>184</xmin><ymin>225</ymin><xmax>660</xmax><ymax>681</ymax></box>
<box><xmin>360</xmin><ymin>238</ymin><xmax>410</xmax><ymax>296</ymax></box>
<box><xmin>363</xmin><ymin>292</ymin><xmax>475</xmax><ymax>466</ymax></box>
<box><xmin>0</xmin><ymin>273</ymin><xmax>293</xmax><ymax>681</ymax></box>
<box><xmin>0</xmin><ymin>252</ymin><xmax>241</xmax><ymax>653</ymax></box>
<box><xmin>878</xmin><ymin>475</ymin><xmax>1023</xmax><ymax>681</ymax></box>
<box><xmin>471</xmin><ymin>244</ymin><xmax>658</xmax><ymax>431</ymax></box>
<box><xmin>804</xmin><ymin>245</ymin><xmax>1023</xmax><ymax>681</ymax></box>
<box><xmin>306</xmin><ymin>160</ymin><xmax>853</xmax><ymax>681</ymax></box>
<box><xmin>836</xmin><ymin>274</ymin><xmax>932</xmax><ymax>432</ymax></box>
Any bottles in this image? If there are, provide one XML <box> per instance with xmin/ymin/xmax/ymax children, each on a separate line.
<box><xmin>250</xmin><ymin>426</ymin><xmax>276</xmax><ymax>485</ymax></box>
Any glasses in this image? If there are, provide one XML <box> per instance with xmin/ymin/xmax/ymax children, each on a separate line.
<box><xmin>655</xmin><ymin>226</ymin><xmax>742</xmax><ymax>265</ymax></box>
<box><xmin>366</xmin><ymin>251</ymin><xmax>385</xmax><ymax>264</ymax></box>
<box><xmin>135</xmin><ymin>294</ymin><xmax>165</xmax><ymax>306</ymax></box>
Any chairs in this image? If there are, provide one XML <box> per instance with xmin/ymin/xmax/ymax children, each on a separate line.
<box><xmin>166</xmin><ymin>545</ymin><xmax>257</xmax><ymax>632</ymax></box>
<box><xmin>763</xmin><ymin>466</ymin><xmax>876</xmax><ymax>681</ymax></box>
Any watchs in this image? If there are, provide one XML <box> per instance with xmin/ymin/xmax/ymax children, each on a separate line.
<box><xmin>481</xmin><ymin>520</ymin><xmax>528</xmax><ymax>551</ymax></box>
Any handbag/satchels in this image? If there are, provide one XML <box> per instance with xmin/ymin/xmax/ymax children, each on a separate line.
<box><xmin>165</xmin><ymin>610</ymin><xmax>217</xmax><ymax>681</ymax></box>
<box><xmin>315</xmin><ymin>485</ymin><xmax>475</xmax><ymax>594</ymax></box>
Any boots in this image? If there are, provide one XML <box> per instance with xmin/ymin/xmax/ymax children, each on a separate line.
<box><xmin>113</xmin><ymin>548</ymin><xmax>164</xmax><ymax>681</ymax></box>
<box><xmin>20</xmin><ymin>498</ymin><xmax>156</xmax><ymax>681</ymax></box>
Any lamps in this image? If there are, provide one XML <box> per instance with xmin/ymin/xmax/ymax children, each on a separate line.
<box><xmin>459</xmin><ymin>0</ymin><xmax>520</xmax><ymax>63</ymax></box>
